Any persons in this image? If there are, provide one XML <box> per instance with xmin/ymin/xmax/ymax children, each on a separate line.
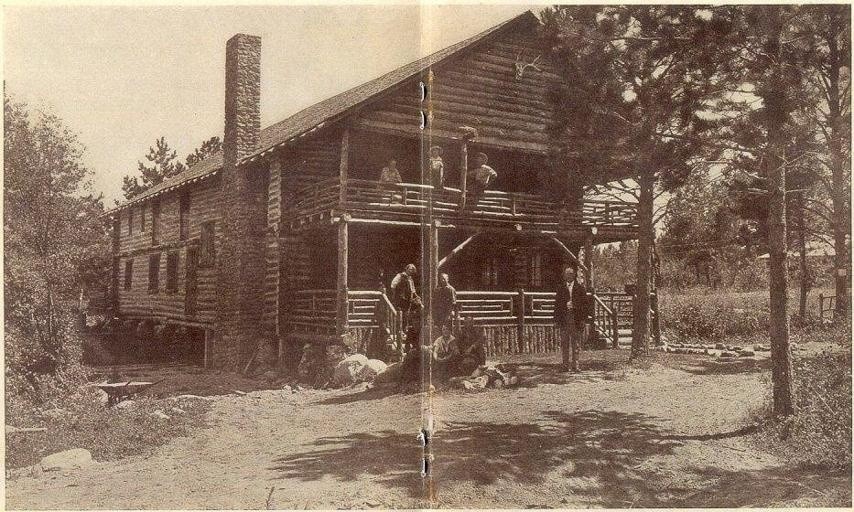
<box><xmin>430</xmin><ymin>144</ymin><xmax>445</xmax><ymax>186</ymax></box>
<box><xmin>466</xmin><ymin>149</ymin><xmax>500</xmax><ymax>190</ymax></box>
<box><xmin>388</xmin><ymin>262</ymin><xmax>423</xmax><ymax>314</ymax></box>
<box><xmin>553</xmin><ymin>267</ymin><xmax>591</xmax><ymax>373</ymax></box>
<box><xmin>401</xmin><ymin>296</ymin><xmax>425</xmax><ymax>352</ymax></box>
<box><xmin>397</xmin><ymin>316</ymin><xmax>433</xmax><ymax>393</ymax></box>
<box><xmin>437</xmin><ymin>272</ymin><xmax>459</xmax><ymax>319</ymax></box>
<box><xmin>459</xmin><ymin>314</ymin><xmax>487</xmax><ymax>366</ymax></box>
<box><xmin>379</xmin><ymin>156</ymin><xmax>407</xmax><ymax>191</ymax></box>
<box><xmin>431</xmin><ymin>323</ymin><xmax>459</xmax><ymax>378</ymax></box>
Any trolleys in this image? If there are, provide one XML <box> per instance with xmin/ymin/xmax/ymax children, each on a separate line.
<box><xmin>95</xmin><ymin>376</ymin><xmax>166</xmax><ymax>404</ymax></box>
